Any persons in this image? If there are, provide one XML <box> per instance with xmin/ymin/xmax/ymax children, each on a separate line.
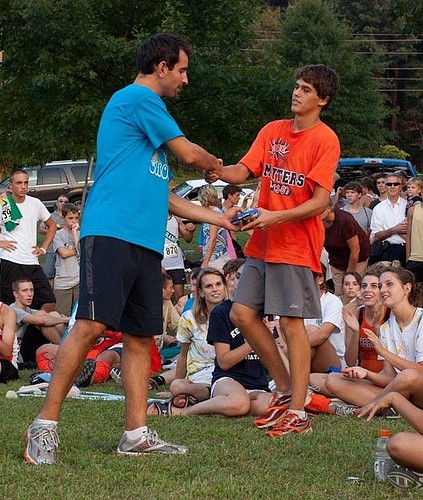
<box><xmin>24</xmin><ymin>32</ymin><xmax>223</xmax><ymax>466</ymax></box>
<box><xmin>355</xmin><ymin>392</ymin><xmax>423</xmax><ymax>490</ymax></box>
<box><xmin>203</xmin><ymin>64</ymin><xmax>341</xmax><ymax>438</ymax></box>
<box><xmin>0</xmin><ymin>169</ymin><xmax>423</xmax><ymax>418</ymax></box>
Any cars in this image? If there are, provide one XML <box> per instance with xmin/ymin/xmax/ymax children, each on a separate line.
<box><xmin>167</xmin><ymin>178</ymin><xmax>255</xmax><ymax>223</ymax></box>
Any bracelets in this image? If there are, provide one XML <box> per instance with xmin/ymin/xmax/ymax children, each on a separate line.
<box><xmin>40</xmin><ymin>247</ymin><xmax>47</xmax><ymax>254</ymax></box>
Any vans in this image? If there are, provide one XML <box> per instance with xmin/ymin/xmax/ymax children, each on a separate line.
<box><xmin>335</xmin><ymin>158</ymin><xmax>418</xmax><ymax>192</ymax></box>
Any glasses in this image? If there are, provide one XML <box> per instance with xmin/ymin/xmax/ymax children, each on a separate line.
<box><xmin>386</xmin><ymin>183</ymin><xmax>401</xmax><ymax>187</ymax></box>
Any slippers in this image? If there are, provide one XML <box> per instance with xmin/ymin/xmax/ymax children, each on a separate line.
<box><xmin>174</xmin><ymin>394</ymin><xmax>199</xmax><ymax>408</ymax></box>
<box><xmin>147</xmin><ymin>400</ymin><xmax>172</xmax><ymax>417</ymax></box>
<box><xmin>149</xmin><ymin>376</ymin><xmax>166</xmax><ymax>389</ymax></box>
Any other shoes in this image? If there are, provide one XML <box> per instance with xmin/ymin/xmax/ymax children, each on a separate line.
<box><xmin>74</xmin><ymin>359</ymin><xmax>95</xmax><ymax>388</ymax></box>
<box><xmin>110</xmin><ymin>367</ymin><xmax>122</xmax><ymax>384</ymax></box>
<box><xmin>386</xmin><ymin>464</ymin><xmax>422</xmax><ymax>491</ymax></box>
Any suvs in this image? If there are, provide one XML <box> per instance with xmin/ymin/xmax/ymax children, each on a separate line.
<box><xmin>1</xmin><ymin>158</ymin><xmax>97</xmax><ymax>216</ymax></box>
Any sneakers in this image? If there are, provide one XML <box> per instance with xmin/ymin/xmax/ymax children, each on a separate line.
<box><xmin>265</xmin><ymin>411</ymin><xmax>313</xmax><ymax>437</ymax></box>
<box><xmin>328</xmin><ymin>398</ymin><xmax>357</xmax><ymax>416</ymax></box>
<box><xmin>117</xmin><ymin>428</ymin><xmax>189</xmax><ymax>455</ymax></box>
<box><xmin>254</xmin><ymin>392</ymin><xmax>291</xmax><ymax>428</ymax></box>
<box><xmin>24</xmin><ymin>418</ymin><xmax>59</xmax><ymax>465</ymax></box>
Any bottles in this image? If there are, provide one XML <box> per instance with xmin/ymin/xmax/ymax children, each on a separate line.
<box><xmin>373</xmin><ymin>430</ymin><xmax>392</xmax><ymax>484</ymax></box>
